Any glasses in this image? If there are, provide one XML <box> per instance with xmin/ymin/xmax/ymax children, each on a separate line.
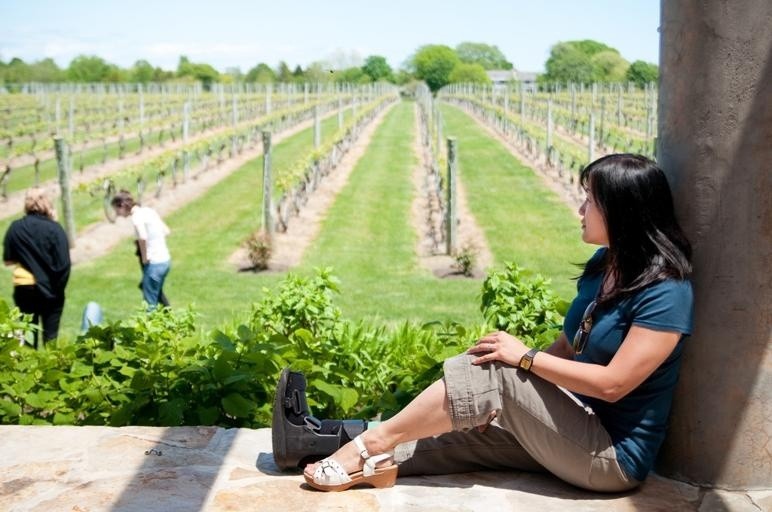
<box><xmin>572</xmin><ymin>299</ymin><xmax>598</xmax><ymax>355</ymax></box>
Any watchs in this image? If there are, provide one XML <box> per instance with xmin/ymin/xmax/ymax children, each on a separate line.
<box><xmin>519</xmin><ymin>348</ymin><xmax>539</xmax><ymax>373</ymax></box>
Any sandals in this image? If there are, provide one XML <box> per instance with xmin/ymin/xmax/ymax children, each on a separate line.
<box><xmin>303</xmin><ymin>434</ymin><xmax>399</xmax><ymax>493</ymax></box>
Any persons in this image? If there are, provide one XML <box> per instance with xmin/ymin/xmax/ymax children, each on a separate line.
<box><xmin>111</xmin><ymin>188</ymin><xmax>172</xmax><ymax>323</ymax></box>
<box><xmin>3</xmin><ymin>187</ymin><xmax>72</xmax><ymax>353</ymax></box>
<box><xmin>271</xmin><ymin>154</ymin><xmax>694</xmax><ymax>492</ymax></box>
